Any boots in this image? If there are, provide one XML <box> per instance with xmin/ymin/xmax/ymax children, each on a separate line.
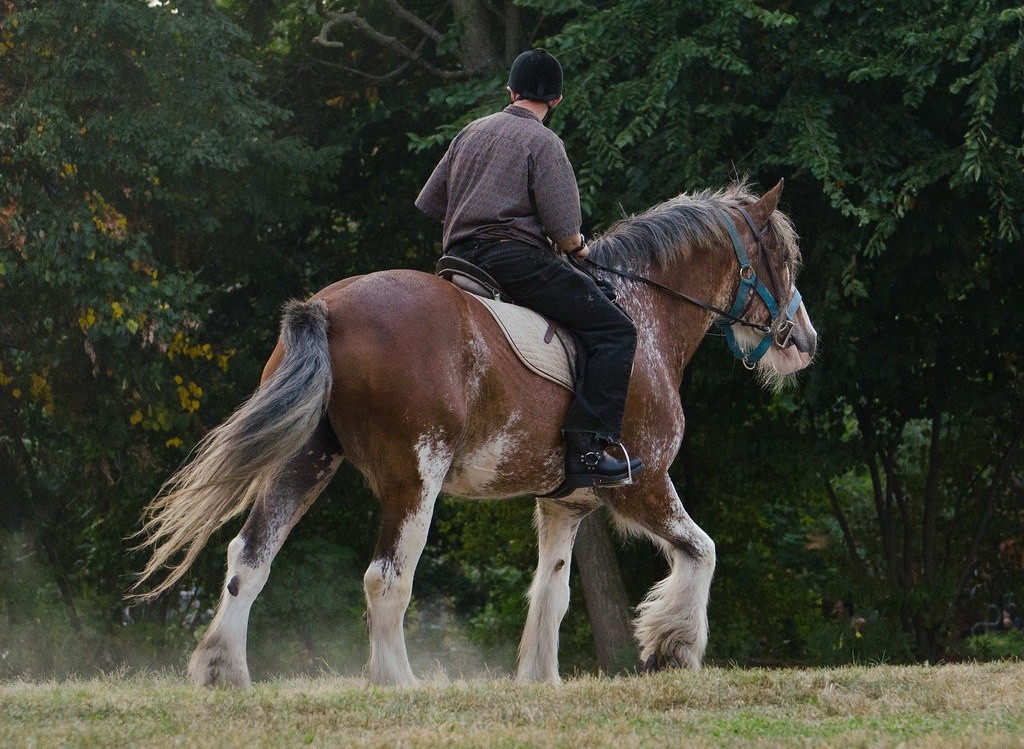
<box><xmin>562</xmin><ymin>429</ymin><xmax>646</xmax><ymax>488</ymax></box>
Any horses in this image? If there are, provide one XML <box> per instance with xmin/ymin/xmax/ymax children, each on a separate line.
<box><xmin>121</xmin><ymin>177</ymin><xmax>818</xmax><ymax>688</ymax></box>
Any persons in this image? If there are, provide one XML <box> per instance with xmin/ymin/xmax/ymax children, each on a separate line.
<box><xmin>414</xmin><ymin>46</ymin><xmax>649</xmax><ymax>486</ymax></box>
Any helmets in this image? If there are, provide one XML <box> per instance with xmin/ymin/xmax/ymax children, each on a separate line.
<box><xmin>508</xmin><ymin>48</ymin><xmax>563</xmax><ymax>100</ymax></box>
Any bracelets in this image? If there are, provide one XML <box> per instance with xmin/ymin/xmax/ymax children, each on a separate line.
<box><xmin>570</xmin><ymin>232</ymin><xmax>586</xmax><ymax>257</ymax></box>
